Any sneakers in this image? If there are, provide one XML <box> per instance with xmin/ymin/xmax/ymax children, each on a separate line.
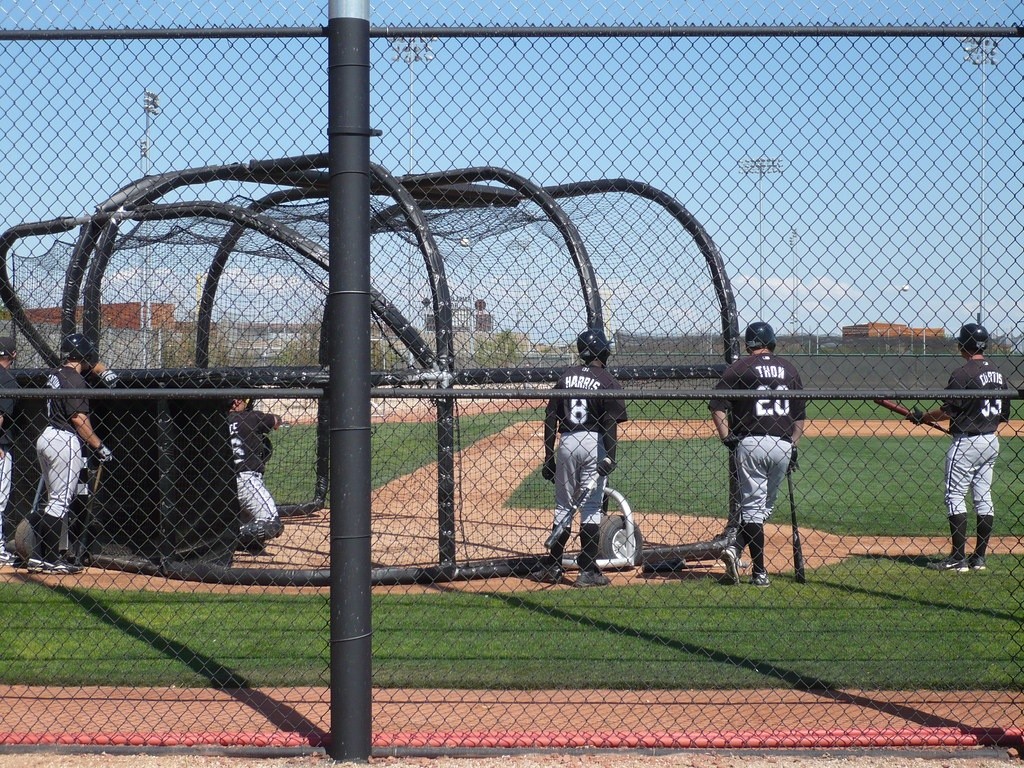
<box><xmin>576</xmin><ymin>570</ymin><xmax>609</xmax><ymax>588</ymax></box>
<box><xmin>927</xmin><ymin>554</ymin><xmax>969</xmax><ymax>572</ymax></box>
<box><xmin>0</xmin><ymin>549</ymin><xmax>18</xmax><ymax>566</ymax></box>
<box><xmin>968</xmin><ymin>553</ymin><xmax>986</xmax><ymax>570</ymax></box>
<box><xmin>42</xmin><ymin>554</ymin><xmax>84</xmax><ymax>574</ymax></box>
<box><xmin>749</xmin><ymin>568</ymin><xmax>771</xmax><ymax>587</ymax></box>
<box><xmin>27</xmin><ymin>558</ymin><xmax>43</xmax><ymax>573</ymax></box>
<box><xmin>721</xmin><ymin>545</ymin><xmax>743</xmax><ymax>584</ymax></box>
<box><xmin>531</xmin><ymin>566</ymin><xmax>564</xmax><ymax>584</ymax></box>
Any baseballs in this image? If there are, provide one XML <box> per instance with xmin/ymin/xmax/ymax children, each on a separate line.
<box><xmin>603</xmin><ymin>457</ymin><xmax>611</xmax><ymax>464</ymax></box>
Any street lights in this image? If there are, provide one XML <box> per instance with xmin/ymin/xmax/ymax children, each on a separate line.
<box><xmin>737</xmin><ymin>157</ymin><xmax>785</xmax><ymax>324</ymax></box>
<box><xmin>959</xmin><ymin>36</ymin><xmax>1000</xmax><ymax>326</ymax></box>
<box><xmin>137</xmin><ymin>88</ymin><xmax>159</xmax><ymax>370</ymax></box>
<box><xmin>390</xmin><ymin>37</ymin><xmax>435</xmax><ymax>372</ymax></box>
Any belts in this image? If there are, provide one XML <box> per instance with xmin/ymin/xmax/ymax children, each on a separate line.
<box><xmin>968</xmin><ymin>431</ymin><xmax>994</xmax><ymax>437</ymax></box>
<box><xmin>243</xmin><ymin>472</ymin><xmax>259</xmax><ymax>477</ymax></box>
<box><xmin>747</xmin><ymin>433</ymin><xmax>792</xmax><ymax>443</ymax></box>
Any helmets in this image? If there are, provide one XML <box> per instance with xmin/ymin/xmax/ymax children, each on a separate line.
<box><xmin>745</xmin><ymin>322</ymin><xmax>776</xmax><ymax>353</ymax></box>
<box><xmin>61</xmin><ymin>333</ymin><xmax>91</xmax><ymax>359</ymax></box>
<box><xmin>577</xmin><ymin>330</ymin><xmax>613</xmax><ymax>361</ymax></box>
<box><xmin>957</xmin><ymin>324</ymin><xmax>988</xmax><ymax>352</ymax></box>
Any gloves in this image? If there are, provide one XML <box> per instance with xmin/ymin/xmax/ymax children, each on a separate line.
<box><xmin>542</xmin><ymin>463</ymin><xmax>557</xmax><ymax>484</ymax></box>
<box><xmin>98</xmin><ymin>444</ymin><xmax>112</xmax><ymax>462</ymax></box>
<box><xmin>723</xmin><ymin>434</ymin><xmax>743</xmax><ymax>454</ymax></box>
<box><xmin>787</xmin><ymin>445</ymin><xmax>799</xmax><ymax>475</ymax></box>
<box><xmin>98</xmin><ymin>369</ymin><xmax>118</xmax><ymax>387</ymax></box>
<box><xmin>596</xmin><ymin>460</ymin><xmax>617</xmax><ymax>476</ymax></box>
<box><xmin>906</xmin><ymin>406</ymin><xmax>925</xmax><ymax>426</ymax></box>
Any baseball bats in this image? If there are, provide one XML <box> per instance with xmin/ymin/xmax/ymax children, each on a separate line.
<box><xmin>873</xmin><ymin>399</ymin><xmax>950</xmax><ymax>435</ymax></box>
<box><xmin>74</xmin><ymin>462</ymin><xmax>104</xmax><ymax>565</ymax></box>
<box><xmin>786</xmin><ymin>468</ymin><xmax>805</xmax><ymax>583</ymax></box>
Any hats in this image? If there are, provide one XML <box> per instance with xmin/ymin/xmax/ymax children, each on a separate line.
<box><xmin>0</xmin><ymin>337</ymin><xmax>16</xmax><ymax>356</ymax></box>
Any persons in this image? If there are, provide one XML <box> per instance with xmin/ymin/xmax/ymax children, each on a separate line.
<box><xmin>0</xmin><ymin>336</ymin><xmax>23</xmax><ymax>566</ymax></box>
<box><xmin>27</xmin><ymin>334</ymin><xmax>118</xmax><ymax>573</ymax></box>
<box><xmin>708</xmin><ymin>322</ymin><xmax>806</xmax><ymax>588</ymax></box>
<box><xmin>230</xmin><ymin>398</ymin><xmax>285</xmax><ymax>547</ymax></box>
<box><xmin>907</xmin><ymin>324</ymin><xmax>1011</xmax><ymax>572</ymax></box>
<box><xmin>532</xmin><ymin>329</ymin><xmax>628</xmax><ymax>587</ymax></box>
